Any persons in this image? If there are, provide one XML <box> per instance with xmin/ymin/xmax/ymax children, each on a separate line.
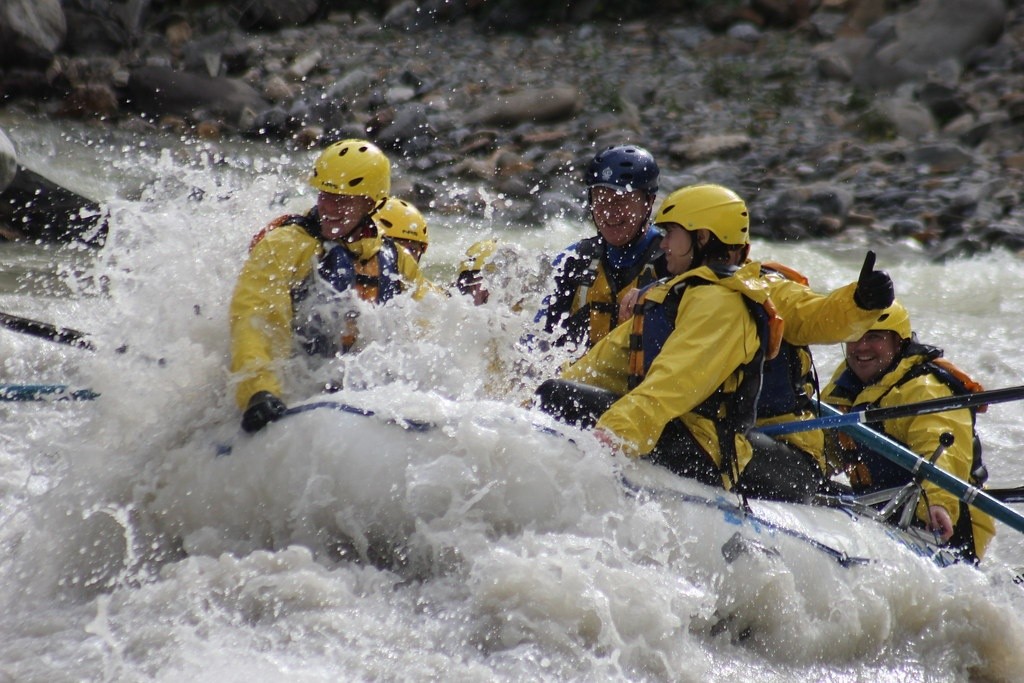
<box><xmin>229</xmin><ymin>139</ymin><xmax>532</xmax><ymax>429</ymax></box>
<box><xmin>818</xmin><ymin>301</ymin><xmax>996</xmax><ymax>566</ymax></box>
<box><xmin>516</xmin><ymin>145</ymin><xmax>895</xmax><ymax>503</ymax></box>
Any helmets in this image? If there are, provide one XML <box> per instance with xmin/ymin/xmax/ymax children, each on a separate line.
<box><xmin>458</xmin><ymin>240</ymin><xmax>501</xmax><ymax>273</ymax></box>
<box><xmin>368</xmin><ymin>194</ymin><xmax>429</xmax><ymax>245</ymax></box>
<box><xmin>307</xmin><ymin>137</ymin><xmax>391</xmax><ymax>202</ymax></box>
<box><xmin>652</xmin><ymin>183</ymin><xmax>750</xmax><ymax>246</ymax></box>
<box><xmin>868</xmin><ymin>300</ymin><xmax>913</xmax><ymax>341</ymax></box>
<box><xmin>585</xmin><ymin>144</ymin><xmax>660</xmax><ymax>198</ymax></box>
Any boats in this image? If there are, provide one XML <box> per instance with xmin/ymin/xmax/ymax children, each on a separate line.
<box><xmin>206</xmin><ymin>382</ymin><xmax>976</xmax><ymax>601</ymax></box>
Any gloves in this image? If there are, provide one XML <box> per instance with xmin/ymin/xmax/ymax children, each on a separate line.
<box><xmin>853</xmin><ymin>251</ymin><xmax>894</xmax><ymax>310</ymax></box>
<box><xmin>242</xmin><ymin>392</ymin><xmax>286</xmax><ymax>433</ymax></box>
